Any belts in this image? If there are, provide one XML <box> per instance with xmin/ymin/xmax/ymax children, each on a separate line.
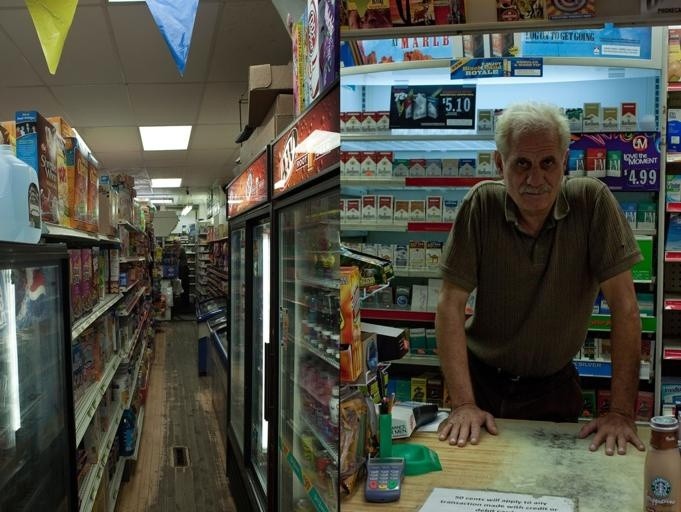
<box><xmin>495</xmin><ymin>368</ymin><xmax>521</xmax><ymax>382</ymax></box>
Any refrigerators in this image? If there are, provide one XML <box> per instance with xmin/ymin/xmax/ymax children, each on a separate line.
<box><xmin>270</xmin><ymin>82</ymin><xmax>341</xmax><ymax>512</ymax></box>
<box><xmin>0</xmin><ymin>243</ymin><xmax>77</xmax><ymax>512</ymax></box>
<box><xmin>224</xmin><ymin>137</ymin><xmax>271</xmax><ymax>507</ymax></box>
<box><xmin>192</xmin><ymin>295</ymin><xmax>229</xmax><ymax>444</ymax></box>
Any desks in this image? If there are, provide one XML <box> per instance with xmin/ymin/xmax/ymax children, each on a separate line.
<box><xmin>340</xmin><ymin>415</ymin><xmax>681</xmax><ymax>512</ymax></box>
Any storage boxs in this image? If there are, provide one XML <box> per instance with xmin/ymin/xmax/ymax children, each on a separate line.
<box><xmin>0</xmin><ymin>108</ymin><xmax>153</xmax><ymax>238</ymax></box>
<box><xmin>230</xmin><ymin>56</ymin><xmax>294</xmax><ymax>184</ymax></box>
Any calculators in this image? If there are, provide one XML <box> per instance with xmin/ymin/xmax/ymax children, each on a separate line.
<box><xmin>365</xmin><ymin>457</ymin><xmax>405</xmax><ymax>502</ymax></box>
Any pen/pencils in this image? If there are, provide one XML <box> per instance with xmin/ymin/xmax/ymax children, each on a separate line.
<box><xmin>377</xmin><ymin>368</ymin><xmax>395</xmax><ymax>415</ymax></box>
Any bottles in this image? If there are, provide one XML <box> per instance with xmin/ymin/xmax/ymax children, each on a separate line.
<box><xmin>291</xmin><ymin>187</ymin><xmax>340</xmax><ymax>510</ymax></box>
<box><xmin>644</xmin><ymin>416</ymin><xmax>681</xmax><ymax>512</ymax></box>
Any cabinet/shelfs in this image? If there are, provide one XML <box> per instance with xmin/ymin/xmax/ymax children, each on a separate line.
<box><xmin>43</xmin><ymin>216</ymin><xmax>230</xmax><ymax>512</ymax></box>
<box><xmin>341</xmin><ymin>17</ymin><xmax>664</xmax><ymax>433</ymax></box>
<box><xmin>659</xmin><ymin>81</ymin><xmax>681</xmax><ymax>415</ymax></box>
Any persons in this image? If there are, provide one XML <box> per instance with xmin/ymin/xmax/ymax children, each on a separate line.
<box><xmin>434</xmin><ymin>102</ymin><xmax>646</xmax><ymax>456</ymax></box>
